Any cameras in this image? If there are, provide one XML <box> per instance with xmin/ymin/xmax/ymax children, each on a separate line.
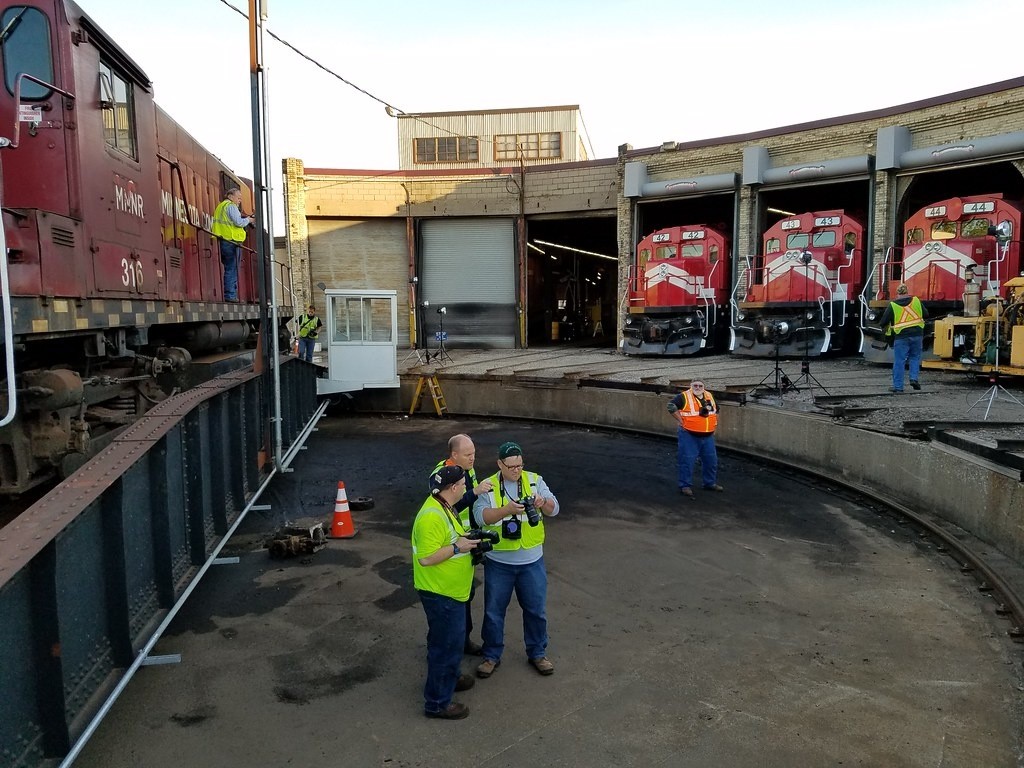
<box><xmin>248</xmin><ymin>214</ymin><xmax>256</xmax><ymax>229</ymax></box>
<box><xmin>517</xmin><ymin>496</ymin><xmax>540</xmax><ymax>527</ymax></box>
<box><xmin>466</xmin><ymin>528</ymin><xmax>500</xmax><ymax>565</ymax></box>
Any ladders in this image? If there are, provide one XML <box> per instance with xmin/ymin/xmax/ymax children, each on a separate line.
<box><xmin>408</xmin><ymin>368</ymin><xmax>450</xmax><ymax>418</ymax></box>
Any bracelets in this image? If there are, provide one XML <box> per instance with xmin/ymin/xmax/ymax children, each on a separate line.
<box><xmin>543</xmin><ymin>497</ymin><xmax>547</xmax><ymax>505</ymax></box>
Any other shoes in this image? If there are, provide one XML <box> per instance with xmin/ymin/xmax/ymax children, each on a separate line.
<box><xmin>910</xmin><ymin>379</ymin><xmax>921</xmax><ymax>390</ymax></box>
<box><xmin>705</xmin><ymin>484</ymin><xmax>723</xmax><ymax>491</ymax></box>
<box><xmin>681</xmin><ymin>487</ymin><xmax>693</xmax><ymax>495</ymax></box>
<box><xmin>888</xmin><ymin>387</ymin><xmax>903</xmax><ymax>392</ymax></box>
<box><xmin>225</xmin><ymin>298</ymin><xmax>240</xmax><ymax>303</ymax></box>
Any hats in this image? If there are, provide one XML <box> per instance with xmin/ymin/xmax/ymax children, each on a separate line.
<box><xmin>436</xmin><ymin>465</ymin><xmax>465</xmax><ymax>487</ymax></box>
<box><xmin>896</xmin><ymin>283</ymin><xmax>908</xmax><ymax>294</ymax></box>
<box><xmin>692</xmin><ymin>377</ymin><xmax>705</xmax><ymax>385</ymax></box>
<box><xmin>498</xmin><ymin>442</ymin><xmax>522</xmax><ymax>459</ymax></box>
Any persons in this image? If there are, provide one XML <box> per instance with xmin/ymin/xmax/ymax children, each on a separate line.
<box><xmin>880</xmin><ymin>285</ymin><xmax>930</xmax><ymax>391</ymax></box>
<box><xmin>429</xmin><ymin>434</ymin><xmax>482</xmax><ymax>656</ymax></box>
<box><xmin>411</xmin><ymin>464</ymin><xmax>481</xmax><ymax>720</ymax></box>
<box><xmin>295</xmin><ymin>304</ymin><xmax>323</xmax><ymax>363</ymax></box>
<box><xmin>210</xmin><ymin>188</ymin><xmax>256</xmax><ymax>302</ymax></box>
<box><xmin>472</xmin><ymin>443</ymin><xmax>559</xmax><ymax>678</ymax></box>
<box><xmin>668</xmin><ymin>377</ymin><xmax>724</xmax><ymax>496</ymax></box>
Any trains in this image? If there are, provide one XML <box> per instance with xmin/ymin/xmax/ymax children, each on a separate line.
<box><xmin>857</xmin><ymin>192</ymin><xmax>1024</xmax><ymax>366</ymax></box>
<box><xmin>730</xmin><ymin>209</ymin><xmax>868</xmax><ymax>359</ymax></box>
<box><xmin>0</xmin><ymin>0</ymin><xmax>270</xmax><ymax>525</ymax></box>
<box><xmin>620</xmin><ymin>224</ymin><xmax>735</xmax><ymax>358</ymax></box>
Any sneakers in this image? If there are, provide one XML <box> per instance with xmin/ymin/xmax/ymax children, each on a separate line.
<box><xmin>464</xmin><ymin>642</ymin><xmax>482</xmax><ymax>657</ymax></box>
<box><xmin>475</xmin><ymin>658</ymin><xmax>501</xmax><ymax>679</ymax></box>
<box><xmin>425</xmin><ymin>701</ymin><xmax>470</xmax><ymax>720</ymax></box>
<box><xmin>454</xmin><ymin>672</ymin><xmax>475</xmax><ymax>691</ymax></box>
<box><xmin>527</xmin><ymin>657</ymin><xmax>554</xmax><ymax>676</ymax></box>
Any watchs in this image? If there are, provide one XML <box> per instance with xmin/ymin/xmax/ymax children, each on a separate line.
<box><xmin>452</xmin><ymin>543</ymin><xmax>460</xmax><ymax>554</ymax></box>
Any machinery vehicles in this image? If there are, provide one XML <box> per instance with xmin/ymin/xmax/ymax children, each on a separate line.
<box><xmin>921</xmin><ymin>263</ymin><xmax>1024</xmax><ymax>378</ymax></box>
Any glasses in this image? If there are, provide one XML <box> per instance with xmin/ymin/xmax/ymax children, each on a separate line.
<box><xmin>692</xmin><ymin>385</ymin><xmax>704</xmax><ymax>388</ymax></box>
<box><xmin>500</xmin><ymin>458</ymin><xmax>523</xmax><ymax>471</ymax></box>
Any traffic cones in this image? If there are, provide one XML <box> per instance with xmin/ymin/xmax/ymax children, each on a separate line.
<box><xmin>326</xmin><ymin>481</ymin><xmax>359</xmax><ymax>539</ymax></box>
